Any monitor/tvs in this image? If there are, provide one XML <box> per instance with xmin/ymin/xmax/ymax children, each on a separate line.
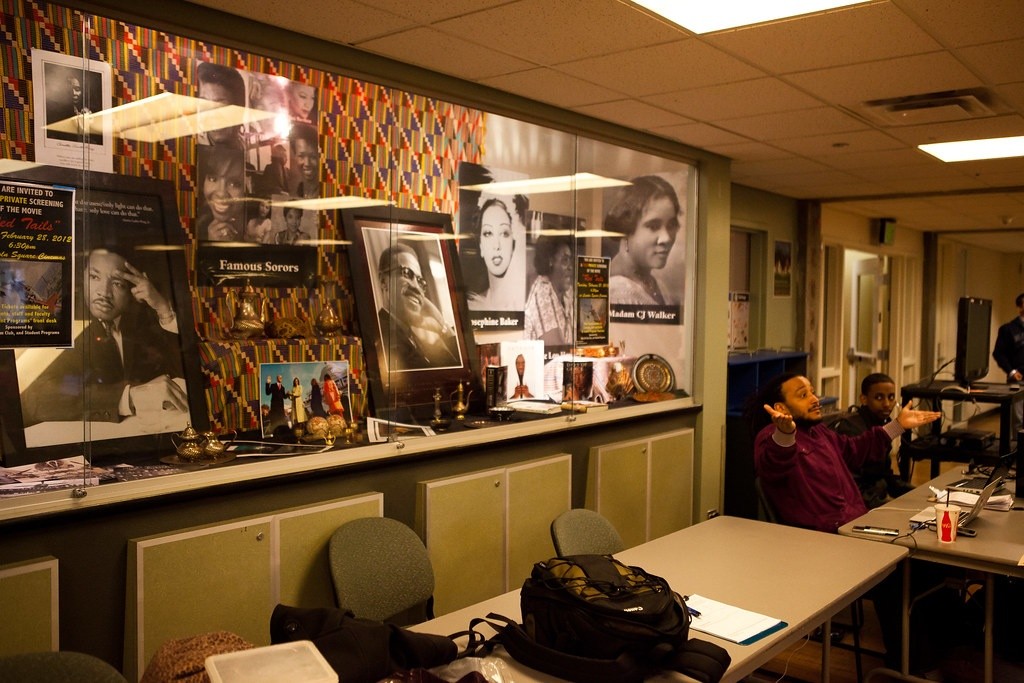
<box><xmin>954</xmin><ymin>296</ymin><xmax>993</xmax><ymax>390</ymax></box>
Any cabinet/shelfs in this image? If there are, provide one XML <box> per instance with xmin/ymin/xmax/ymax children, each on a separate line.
<box><xmin>726</xmin><ymin>351</ymin><xmax>813</xmax><ymax>522</ymax></box>
<box><xmin>412</xmin><ymin>453</ymin><xmax>573</xmax><ymax>617</ymax></box>
<box><xmin>583</xmin><ymin>426</ymin><xmax>695</xmax><ymax>552</ymax></box>
<box><xmin>0</xmin><ymin>553</ymin><xmax>60</xmax><ymax>657</ymax></box>
<box><xmin>899</xmin><ymin>378</ymin><xmax>1024</xmax><ymax>499</ymax></box>
<box><xmin>123</xmin><ymin>489</ymin><xmax>385</xmax><ymax>683</ymax></box>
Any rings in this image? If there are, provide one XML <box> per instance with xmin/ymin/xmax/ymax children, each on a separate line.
<box><xmin>220</xmin><ymin>229</ymin><xmax>227</xmax><ymax>237</ymax></box>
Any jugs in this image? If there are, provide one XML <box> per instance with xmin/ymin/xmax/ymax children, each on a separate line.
<box><xmin>201</xmin><ymin>428</ymin><xmax>237</xmax><ymax>460</ymax></box>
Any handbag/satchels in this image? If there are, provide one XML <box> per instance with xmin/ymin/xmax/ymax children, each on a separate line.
<box><xmin>271</xmin><ymin>603</ymin><xmax>484</xmax><ymax>683</ymax></box>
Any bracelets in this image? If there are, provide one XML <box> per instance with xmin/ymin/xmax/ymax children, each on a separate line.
<box><xmin>777</xmin><ymin>425</ymin><xmax>796</xmax><ymax>435</ymax></box>
<box><xmin>159</xmin><ymin>302</ymin><xmax>177</xmax><ymax>320</ymax></box>
<box><xmin>437</xmin><ymin>320</ymin><xmax>449</xmax><ymax>336</ymax></box>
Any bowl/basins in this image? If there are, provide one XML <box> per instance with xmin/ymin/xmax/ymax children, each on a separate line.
<box><xmin>488</xmin><ymin>406</ymin><xmax>515</xmax><ymax>421</ymax></box>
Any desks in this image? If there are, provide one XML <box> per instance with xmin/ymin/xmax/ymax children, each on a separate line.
<box><xmin>406</xmin><ymin>512</ymin><xmax>909</xmax><ymax>683</ymax></box>
<box><xmin>839</xmin><ymin>465</ymin><xmax>1024</xmax><ymax>683</ymax></box>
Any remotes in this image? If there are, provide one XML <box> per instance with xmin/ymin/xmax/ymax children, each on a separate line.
<box><xmin>1010</xmin><ymin>384</ymin><xmax>1020</xmax><ymax>391</ymax></box>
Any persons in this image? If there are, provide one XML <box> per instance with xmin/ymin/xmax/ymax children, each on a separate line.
<box><xmin>992</xmin><ymin>293</ymin><xmax>1024</xmax><ymax>450</ymax></box>
<box><xmin>262</xmin><ymin>373</ymin><xmax>345</xmax><ymax>434</ymax></box>
<box><xmin>835</xmin><ymin>374</ymin><xmax>966</xmax><ymax>647</ymax></box>
<box><xmin>608</xmin><ymin>174</ymin><xmax>681</xmax><ymax>304</ymax></box>
<box><xmin>469</xmin><ymin>185</ymin><xmax>525</xmax><ymax>313</ymax></box>
<box><xmin>21</xmin><ymin>233</ymin><xmax>190</xmax><ymax>427</ymax></box>
<box><xmin>563</xmin><ymin>364</ymin><xmax>590</xmax><ymax>402</ymax></box>
<box><xmin>377</xmin><ymin>243</ymin><xmax>462</xmax><ymax>371</ymax></box>
<box><xmin>59</xmin><ymin>73</ymin><xmax>101</xmax><ymax>145</ymax></box>
<box><xmin>524</xmin><ymin>235</ymin><xmax>575</xmax><ymax>346</ymax></box>
<box><xmin>197</xmin><ymin>61</ymin><xmax>321</xmax><ymax>243</ymax></box>
<box><xmin>753</xmin><ymin>371</ymin><xmax>961</xmax><ymax>683</ymax></box>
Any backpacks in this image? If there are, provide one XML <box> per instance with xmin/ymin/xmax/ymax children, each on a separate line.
<box><xmin>497</xmin><ymin>553</ymin><xmax>731</xmax><ymax>683</ymax></box>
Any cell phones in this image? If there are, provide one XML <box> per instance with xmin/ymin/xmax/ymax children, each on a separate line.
<box><xmin>852</xmin><ymin>525</ymin><xmax>899</xmax><ymax>536</ymax></box>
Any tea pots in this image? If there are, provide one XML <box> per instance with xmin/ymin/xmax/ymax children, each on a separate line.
<box><xmin>449</xmin><ymin>379</ymin><xmax>473</xmax><ymax>423</ymax></box>
<box><xmin>225</xmin><ymin>278</ymin><xmax>266</xmax><ymax>339</ymax></box>
<box><xmin>171</xmin><ymin>421</ymin><xmax>209</xmax><ymax>462</ymax></box>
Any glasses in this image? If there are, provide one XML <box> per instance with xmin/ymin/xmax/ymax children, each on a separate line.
<box><xmin>384</xmin><ymin>265</ymin><xmax>427</xmax><ymax>293</ymax></box>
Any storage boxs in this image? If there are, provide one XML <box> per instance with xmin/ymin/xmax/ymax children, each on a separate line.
<box><xmin>203</xmin><ymin>640</ymin><xmax>340</xmax><ymax>683</ymax></box>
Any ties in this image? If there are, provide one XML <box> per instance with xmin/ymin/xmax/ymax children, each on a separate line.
<box><xmin>103</xmin><ymin>320</ymin><xmax>114</xmax><ymax>337</ymax></box>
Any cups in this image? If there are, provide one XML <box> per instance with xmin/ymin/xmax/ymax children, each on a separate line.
<box><xmin>934</xmin><ymin>504</ymin><xmax>962</xmax><ymax>544</ymax></box>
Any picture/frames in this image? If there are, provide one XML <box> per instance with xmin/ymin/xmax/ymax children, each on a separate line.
<box><xmin>0</xmin><ymin>165</ymin><xmax>212</xmax><ymax>470</ymax></box>
<box><xmin>340</xmin><ymin>204</ymin><xmax>485</xmax><ymax>422</ymax></box>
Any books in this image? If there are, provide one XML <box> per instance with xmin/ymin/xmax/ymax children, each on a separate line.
<box><xmin>562</xmin><ymin>400</ymin><xmax>608</xmax><ymax>413</ymax></box>
<box><xmin>508</xmin><ymin>401</ymin><xmax>561</xmax><ymax>414</ymax></box>
<box><xmin>0</xmin><ymin>455</ymin><xmax>100</xmax><ymax>495</ymax></box>
<box><xmin>485</xmin><ymin>366</ymin><xmax>507</xmax><ymax>416</ymax></box>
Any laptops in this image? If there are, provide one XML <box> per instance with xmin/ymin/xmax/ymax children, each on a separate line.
<box><xmin>908</xmin><ymin>476</ymin><xmax>1003</xmax><ymax>526</ymax></box>
<box><xmin>946</xmin><ymin>450</ymin><xmax>1018</xmax><ymax>493</ymax></box>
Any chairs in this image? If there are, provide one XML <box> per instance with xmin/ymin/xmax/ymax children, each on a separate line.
<box><xmin>0</xmin><ymin>650</ymin><xmax>130</xmax><ymax>683</ymax></box>
<box><xmin>326</xmin><ymin>516</ymin><xmax>437</xmax><ymax>629</ymax></box>
<box><xmin>138</xmin><ymin>628</ymin><xmax>257</xmax><ymax>683</ymax></box>
<box><xmin>550</xmin><ymin>507</ymin><xmax>623</xmax><ymax>559</ymax></box>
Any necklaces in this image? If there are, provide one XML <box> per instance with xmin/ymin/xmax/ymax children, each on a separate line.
<box><xmin>639</xmin><ymin>274</ymin><xmax>657</xmax><ymax>296</ymax></box>
